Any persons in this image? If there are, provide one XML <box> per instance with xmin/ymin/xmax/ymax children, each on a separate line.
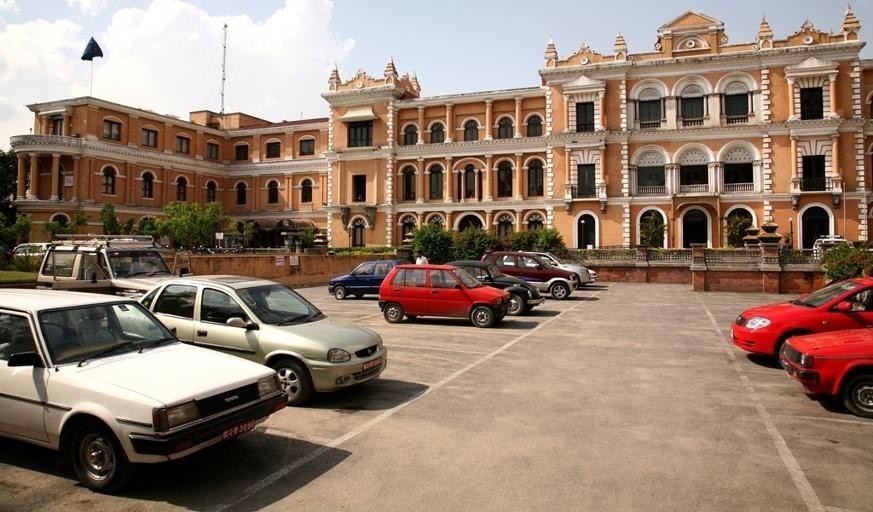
<box><xmin>416</xmin><ymin>250</ymin><xmax>429</xmax><ymax>265</ymax></box>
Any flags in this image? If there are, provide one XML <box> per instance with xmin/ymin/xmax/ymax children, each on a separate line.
<box><xmin>81</xmin><ymin>36</ymin><xmax>104</xmax><ymax>62</ymax></box>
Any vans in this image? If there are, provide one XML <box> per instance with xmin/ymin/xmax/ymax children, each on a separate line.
<box><xmin>8</xmin><ymin>242</ymin><xmax>64</xmax><ymax>267</ymax></box>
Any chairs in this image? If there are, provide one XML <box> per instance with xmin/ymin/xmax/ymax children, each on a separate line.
<box><xmin>495</xmin><ymin>256</ymin><xmax>504</xmax><ymax>268</ymax></box>
<box><xmin>431</xmin><ymin>274</ymin><xmax>446</xmax><ymax>288</ymax></box>
<box><xmin>44</xmin><ymin>325</ymin><xmax>64</xmax><ymax>346</ymax></box>
<box><xmin>75</xmin><ymin>319</ymin><xmax>114</xmax><ymax>345</ymax></box>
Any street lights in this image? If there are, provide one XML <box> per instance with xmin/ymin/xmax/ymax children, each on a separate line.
<box><xmin>788</xmin><ymin>217</ymin><xmax>793</xmax><ymax>249</ymax></box>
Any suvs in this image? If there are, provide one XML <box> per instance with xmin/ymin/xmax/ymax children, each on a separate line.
<box><xmin>479</xmin><ymin>251</ymin><xmax>577</xmax><ymax>300</ymax></box>
<box><xmin>812</xmin><ymin>235</ymin><xmax>847</xmax><ymax>259</ymax></box>
<box><xmin>34</xmin><ymin>232</ymin><xmax>193</xmax><ymax>298</ymax></box>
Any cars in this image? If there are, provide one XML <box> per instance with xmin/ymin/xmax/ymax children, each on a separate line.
<box><xmin>328</xmin><ymin>260</ymin><xmax>410</xmax><ymax>299</ymax></box>
<box><xmin>117</xmin><ymin>273</ymin><xmax>388</xmax><ymax>404</ymax></box>
<box><xmin>502</xmin><ymin>250</ymin><xmax>589</xmax><ymax>285</ymax></box>
<box><xmin>729</xmin><ymin>275</ymin><xmax>867</xmax><ymax>366</ymax></box>
<box><xmin>526</xmin><ymin>256</ymin><xmax>597</xmax><ymax>282</ymax></box>
<box><xmin>377</xmin><ymin>264</ymin><xmax>511</xmax><ymax>328</ymax></box>
<box><xmin>778</xmin><ymin>327</ymin><xmax>872</xmax><ymax>418</ymax></box>
<box><xmin>0</xmin><ymin>284</ymin><xmax>290</xmax><ymax>492</ymax></box>
<box><xmin>445</xmin><ymin>261</ymin><xmax>544</xmax><ymax>316</ymax></box>
